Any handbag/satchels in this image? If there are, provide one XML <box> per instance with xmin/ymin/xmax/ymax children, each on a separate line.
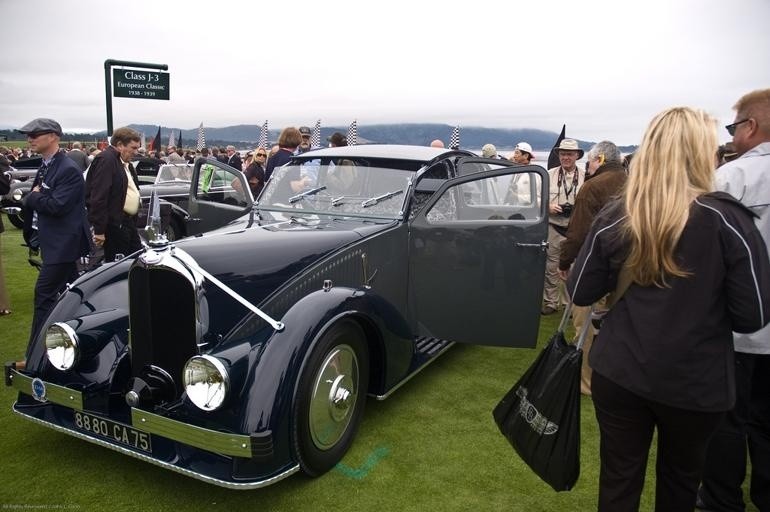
<box><xmin>231</xmin><ymin>162</ymin><xmax>259</xmax><ymax>197</ymax></box>
<box><xmin>492</xmin><ymin>299</ymin><xmax>594</xmax><ymax>492</ymax></box>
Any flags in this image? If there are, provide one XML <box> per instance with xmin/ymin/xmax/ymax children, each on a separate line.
<box><xmin>140</xmin><ymin>122</ymin><xmax>203</xmax><ymax>150</ymax></box>
<box><xmin>258</xmin><ymin>120</ymin><xmax>268</xmax><ymax>147</ymax></box>
<box><xmin>311</xmin><ymin>119</ymin><xmax>357</xmax><ymax>146</ymax></box>
<box><xmin>448</xmin><ymin>127</ymin><xmax>459</xmax><ymax>149</ymax></box>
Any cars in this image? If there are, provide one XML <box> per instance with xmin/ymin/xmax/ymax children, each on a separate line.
<box><xmin>4</xmin><ymin>143</ymin><xmax>551</xmax><ymax>492</ymax></box>
<box><xmin>0</xmin><ymin>151</ymin><xmax>266</xmax><ymax>246</ymax></box>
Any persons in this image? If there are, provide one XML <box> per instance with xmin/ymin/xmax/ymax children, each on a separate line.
<box><xmin>0</xmin><ymin>118</ymin><xmax>141</xmax><ymax>371</ymax></box>
<box><xmin>566</xmin><ymin>107</ymin><xmax>769</xmax><ymax>511</ymax></box>
<box><xmin>558</xmin><ymin>141</ymin><xmax>626</xmax><ymax>394</ymax></box>
<box><xmin>472</xmin><ymin>139</ymin><xmax>585</xmax><ymax>313</ymax></box>
<box><xmin>432</xmin><ymin>139</ymin><xmax>444</xmax><ymax>148</ymax></box>
<box><xmin>244</xmin><ymin>126</ymin><xmax>358</xmax><ymax>206</ymax></box>
<box><xmin>139</xmin><ymin>144</ymin><xmax>251</xmax><ymax>179</ymax></box>
<box><xmin>697</xmin><ymin>88</ymin><xmax>769</xmax><ymax>511</ymax></box>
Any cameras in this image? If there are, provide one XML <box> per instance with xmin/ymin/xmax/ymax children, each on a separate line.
<box><xmin>558</xmin><ymin>202</ymin><xmax>573</xmax><ymax>218</ymax></box>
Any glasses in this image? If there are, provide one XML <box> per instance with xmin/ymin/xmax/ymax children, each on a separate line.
<box><xmin>257</xmin><ymin>153</ymin><xmax>267</xmax><ymax>158</ymax></box>
<box><xmin>27</xmin><ymin>132</ymin><xmax>53</xmax><ymax>140</ymax></box>
<box><xmin>725</xmin><ymin>119</ymin><xmax>749</xmax><ymax>136</ymax></box>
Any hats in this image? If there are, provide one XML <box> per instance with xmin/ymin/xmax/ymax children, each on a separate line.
<box><xmin>481</xmin><ymin>143</ymin><xmax>498</xmax><ymax>158</ymax></box>
<box><xmin>553</xmin><ymin>138</ymin><xmax>584</xmax><ymax>160</ymax></box>
<box><xmin>326</xmin><ymin>132</ymin><xmax>347</xmax><ymax>146</ymax></box>
<box><xmin>515</xmin><ymin>142</ymin><xmax>535</xmax><ymax>158</ymax></box>
<box><xmin>299</xmin><ymin>127</ymin><xmax>311</xmax><ymax>137</ymax></box>
<box><xmin>225</xmin><ymin>146</ymin><xmax>236</xmax><ymax>152</ymax></box>
<box><xmin>18</xmin><ymin>118</ymin><xmax>61</xmax><ymax>136</ymax></box>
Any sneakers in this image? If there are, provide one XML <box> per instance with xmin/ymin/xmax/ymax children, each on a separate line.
<box><xmin>0</xmin><ymin>309</ymin><xmax>11</xmax><ymax>315</ymax></box>
<box><xmin>540</xmin><ymin>307</ymin><xmax>556</xmax><ymax>316</ymax></box>
